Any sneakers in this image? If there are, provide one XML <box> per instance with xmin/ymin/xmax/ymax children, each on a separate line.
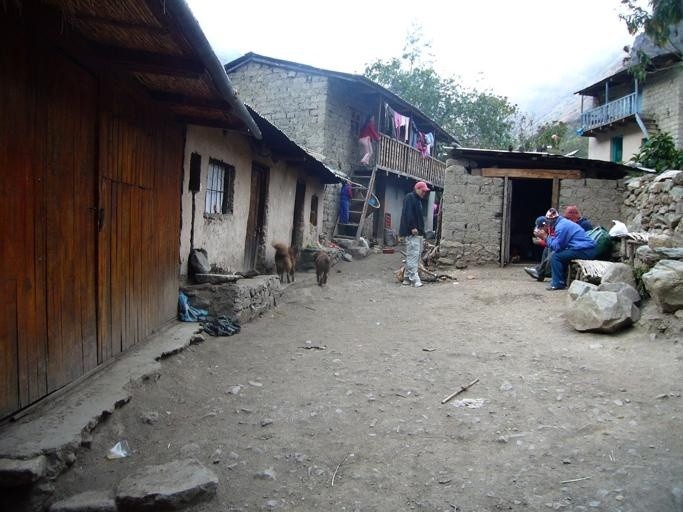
<box><xmin>402</xmin><ymin>278</ymin><xmax>424</xmax><ymax>288</ymax></box>
<box><xmin>544</xmin><ymin>284</ymin><xmax>566</xmax><ymax>290</ymax></box>
<box><xmin>524</xmin><ymin>267</ymin><xmax>545</xmax><ymax>281</ymax></box>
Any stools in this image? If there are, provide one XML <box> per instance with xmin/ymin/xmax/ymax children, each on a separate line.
<box><xmin>565</xmin><ymin>260</ymin><xmax>586</xmax><ymax>287</ymax></box>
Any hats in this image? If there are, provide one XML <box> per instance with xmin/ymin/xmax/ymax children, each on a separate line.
<box><xmin>563</xmin><ymin>205</ymin><xmax>581</xmax><ymax>221</ymax></box>
<box><xmin>535</xmin><ymin>216</ymin><xmax>548</xmax><ymax>228</ymax></box>
<box><xmin>415</xmin><ymin>181</ymin><xmax>431</xmax><ymax>192</ymax></box>
<box><xmin>544</xmin><ymin>208</ymin><xmax>559</xmax><ymax>220</ymax></box>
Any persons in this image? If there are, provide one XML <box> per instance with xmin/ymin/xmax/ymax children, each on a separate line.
<box><xmin>359</xmin><ymin>111</ymin><xmax>382</xmax><ymax>166</ymax></box>
<box><xmin>523</xmin><ymin>205</ymin><xmax>593</xmax><ymax>283</ymax></box>
<box><xmin>339</xmin><ymin>176</ymin><xmax>353</xmax><ymax>225</ymax></box>
<box><xmin>398</xmin><ymin>180</ymin><xmax>432</xmax><ymax>287</ymax></box>
<box><xmin>532</xmin><ymin>213</ymin><xmax>596</xmax><ymax>292</ymax></box>
<box><xmin>539</xmin><ymin>206</ymin><xmax>559</xmax><ymax>263</ymax></box>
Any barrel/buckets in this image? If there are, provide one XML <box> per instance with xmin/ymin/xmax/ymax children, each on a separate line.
<box><xmin>351</xmin><ymin>186</ymin><xmax>381</xmax><ymax>222</ymax></box>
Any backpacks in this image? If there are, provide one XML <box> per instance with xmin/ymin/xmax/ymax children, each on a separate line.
<box><xmin>585</xmin><ymin>226</ymin><xmax>612</xmax><ymax>259</ymax></box>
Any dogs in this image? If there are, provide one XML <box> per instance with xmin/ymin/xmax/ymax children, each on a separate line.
<box><xmin>269</xmin><ymin>240</ymin><xmax>300</xmax><ymax>284</ymax></box>
<box><xmin>311</xmin><ymin>251</ymin><xmax>332</xmax><ymax>286</ymax></box>
